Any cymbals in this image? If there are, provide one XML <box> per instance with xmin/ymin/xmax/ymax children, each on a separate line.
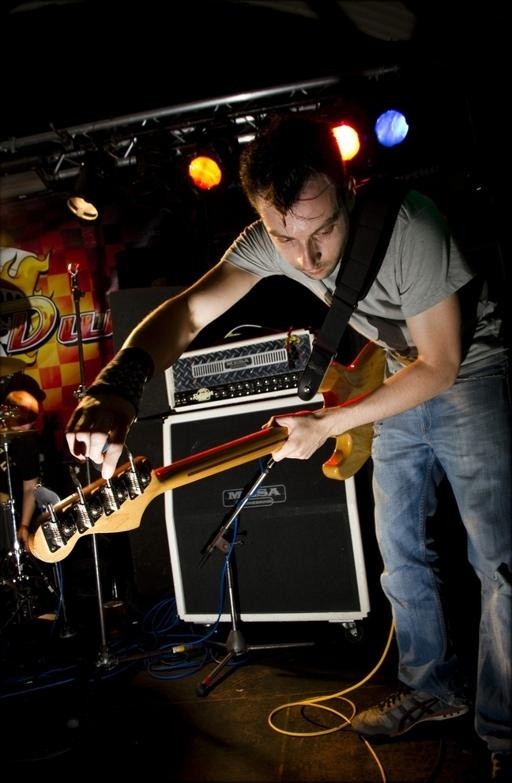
<box><xmin>0</xmin><ymin>400</ymin><xmax>37</xmax><ymax>427</ymax></box>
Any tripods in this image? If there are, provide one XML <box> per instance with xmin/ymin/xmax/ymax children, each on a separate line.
<box><xmin>198</xmin><ymin>544</ymin><xmax>315</xmax><ymax>695</ymax></box>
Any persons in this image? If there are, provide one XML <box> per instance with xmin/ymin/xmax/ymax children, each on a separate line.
<box><xmin>60</xmin><ymin>113</ymin><xmax>512</xmax><ymax>781</ymax></box>
<box><xmin>1</xmin><ymin>388</ymin><xmax>66</xmax><ymax>555</ymax></box>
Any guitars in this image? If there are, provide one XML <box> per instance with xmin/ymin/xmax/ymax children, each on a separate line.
<box><xmin>21</xmin><ymin>341</ymin><xmax>384</xmax><ymax>566</ymax></box>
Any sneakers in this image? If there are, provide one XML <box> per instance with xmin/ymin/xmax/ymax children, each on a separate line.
<box><xmin>352</xmin><ymin>685</ymin><xmax>469</xmax><ymax>736</ymax></box>
<box><xmin>492</xmin><ymin>749</ymin><xmax>512</xmax><ymax>783</ymax></box>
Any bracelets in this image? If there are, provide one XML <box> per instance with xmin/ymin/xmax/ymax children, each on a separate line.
<box><xmin>20</xmin><ymin>524</ymin><xmax>29</xmax><ymax>529</ymax></box>
<box><xmin>83</xmin><ymin>345</ymin><xmax>155</xmax><ymax>421</ymax></box>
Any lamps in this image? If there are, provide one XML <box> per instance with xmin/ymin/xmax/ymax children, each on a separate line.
<box><xmin>63</xmin><ymin>140</ymin><xmax>230</xmax><ymax>222</ymax></box>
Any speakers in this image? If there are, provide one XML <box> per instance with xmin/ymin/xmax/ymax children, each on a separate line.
<box><xmin>161</xmin><ymin>392</ymin><xmax>372</xmax><ymax>638</ymax></box>
